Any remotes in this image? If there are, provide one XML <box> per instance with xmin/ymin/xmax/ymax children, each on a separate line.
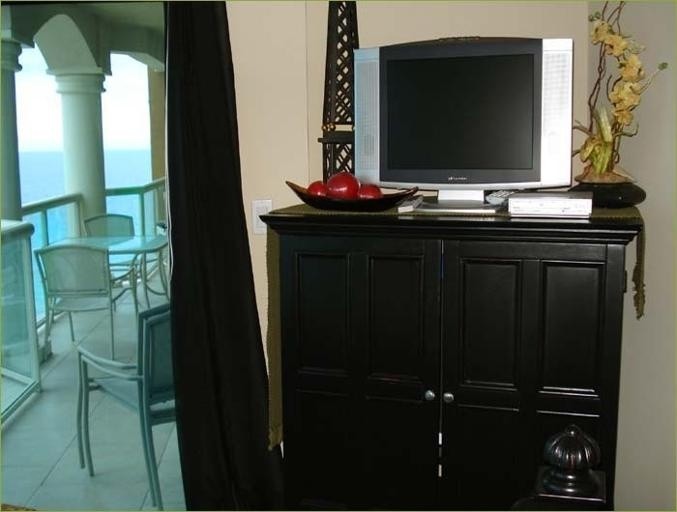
<box><xmin>397</xmin><ymin>193</ymin><xmax>424</xmax><ymax>213</ymax></box>
<box><xmin>485</xmin><ymin>189</ymin><xmax>515</xmax><ymax>206</ymax></box>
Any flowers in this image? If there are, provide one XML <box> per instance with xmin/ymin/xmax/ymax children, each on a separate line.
<box><xmin>572</xmin><ymin>0</ymin><xmax>668</xmax><ymax>177</ymax></box>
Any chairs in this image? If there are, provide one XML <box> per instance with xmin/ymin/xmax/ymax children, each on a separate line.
<box><xmin>75</xmin><ymin>302</ymin><xmax>177</xmax><ymax>512</ymax></box>
<box><xmin>34</xmin><ymin>213</ymin><xmax>139</xmax><ymax>360</ymax></box>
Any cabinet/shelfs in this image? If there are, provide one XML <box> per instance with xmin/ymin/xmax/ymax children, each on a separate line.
<box><xmin>259</xmin><ymin>204</ymin><xmax>644</xmax><ymax>511</ymax></box>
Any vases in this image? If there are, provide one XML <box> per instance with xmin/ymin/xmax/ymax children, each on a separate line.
<box><xmin>571</xmin><ymin>171</ymin><xmax>647</xmax><ymax>208</ymax></box>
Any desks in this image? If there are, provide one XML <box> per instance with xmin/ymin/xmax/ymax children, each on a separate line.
<box><xmin>44</xmin><ymin>236</ymin><xmax>169</xmax><ymax>340</ymax></box>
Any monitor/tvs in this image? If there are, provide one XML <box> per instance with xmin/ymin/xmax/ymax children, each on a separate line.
<box><xmin>352</xmin><ymin>35</ymin><xmax>575</xmax><ymax>214</ymax></box>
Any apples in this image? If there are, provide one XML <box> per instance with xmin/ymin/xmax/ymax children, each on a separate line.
<box><xmin>307</xmin><ymin>170</ymin><xmax>383</xmax><ymax>200</ymax></box>
